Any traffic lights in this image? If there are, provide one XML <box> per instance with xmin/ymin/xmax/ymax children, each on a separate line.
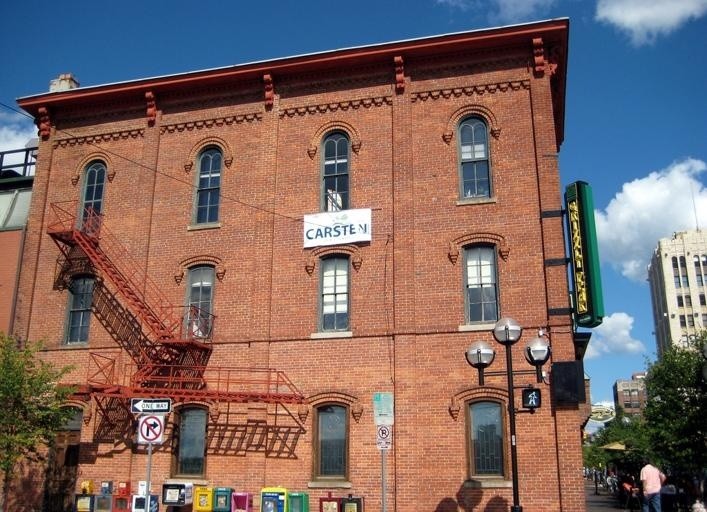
<box><xmin>522</xmin><ymin>388</ymin><xmax>542</xmax><ymax>409</ymax></box>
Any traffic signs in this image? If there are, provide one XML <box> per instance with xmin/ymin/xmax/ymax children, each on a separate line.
<box><xmin>131</xmin><ymin>398</ymin><xmax>172</xmax><ymax>413</ymax></box>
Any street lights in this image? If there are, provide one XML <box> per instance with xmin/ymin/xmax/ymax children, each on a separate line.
<box><xmin>464</xmin><ymin>314</ymin><xmax>554</xmax><ymax>512</ymax></box>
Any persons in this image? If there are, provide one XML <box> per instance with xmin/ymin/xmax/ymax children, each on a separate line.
<box><xmin>584</xmin><ymin>460</ymin><xmax>707</xmax><ymax>511</ymax></box>
